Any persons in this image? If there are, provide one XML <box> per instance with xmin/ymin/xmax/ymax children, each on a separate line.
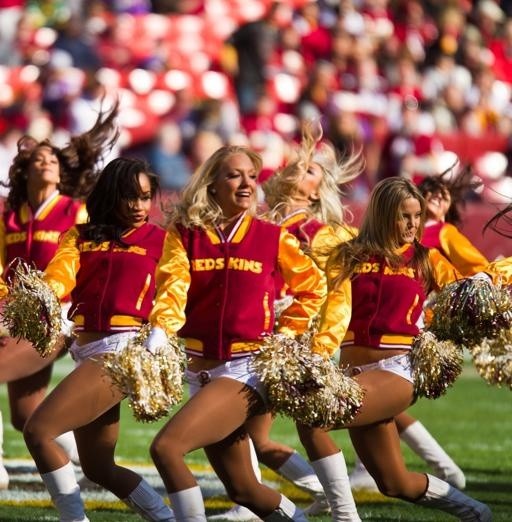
<box><xmin>0</xmin><ymin>0</ymin><xmax>512</xmax><ymax>206</ymax></box>
<box><xmin>146</xmin><ymin>147</ymin><xmax>326</xmax><ymax>521</ymax></box>
<box><xmin>297</xmin><ymin>176</ymin><xmax>493</xmax><ymax>522</ymax></box>
<box><xmin>23</xmin><ymin>155</ymin><xmax>177</xmax><ymax>521</ymax></box>
<box><xmin>348</xmin><ymin>177</ymin><xmax>491</xmax><ymax>493</ymax></box>
<box><xmin>244</xmin><ymin>160</ymin><xmax>360</xmax><ymax>521</ymax></box>
<box><xmin>0</xmin><ymin>139</ymin><xmax>90</xmax><ymax>521</ymax></box>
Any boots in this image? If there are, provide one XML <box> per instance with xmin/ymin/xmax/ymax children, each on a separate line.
<box><xmin>399</xmin><ymin>420</ymin><xmax>466</xmax><ymax>490</ymax></box>
<box><xmin>52</xmin><ymin>429</ymin><xmax>80</xmax><ymax>465</ymax></box>
<box><xmin>207</xmin><ymin>438</ymin><xmax>261</xmax><ymax>521</ymax></box>
<box><xmin>275</xmin><ymin>449</ymin><xmax>332</xmax><ymax>515</ymax></box>
<box><xmin>0</xmin><ymin>410</ymin><xmax>10</xmax><ymax>488</ymax></box>
<box><xmin>348</xmin><ymin>456</ymin><xmax>376</xmax><ymax>489</ymax></box>
<box><xmin>121</xmin><ymin>479</ymin><xmax>176</xmax><ymax>522</ymax></box>
<box><xmin>168</xmin><ymin>486</ymin><xmax>206</xmax><ymax>522</ymax></box>
<box><xmin>414</xmin><ymin>473</ymin><xmax>495</xmax><ymax>522</ymax></box>
<box><xmin>308</xmin><ymin>448</ymin><xmax>362</xmax><ymax>522</ymax></box>
<box><xmin>40</xmin><ymin>460</ymin><xmax>89</xmax><ymax>522</ymax></box>
<box><xmin>263</xmin><ymin>493</ymin><xmax>308</xmax><ymax>522</ymax></box>
